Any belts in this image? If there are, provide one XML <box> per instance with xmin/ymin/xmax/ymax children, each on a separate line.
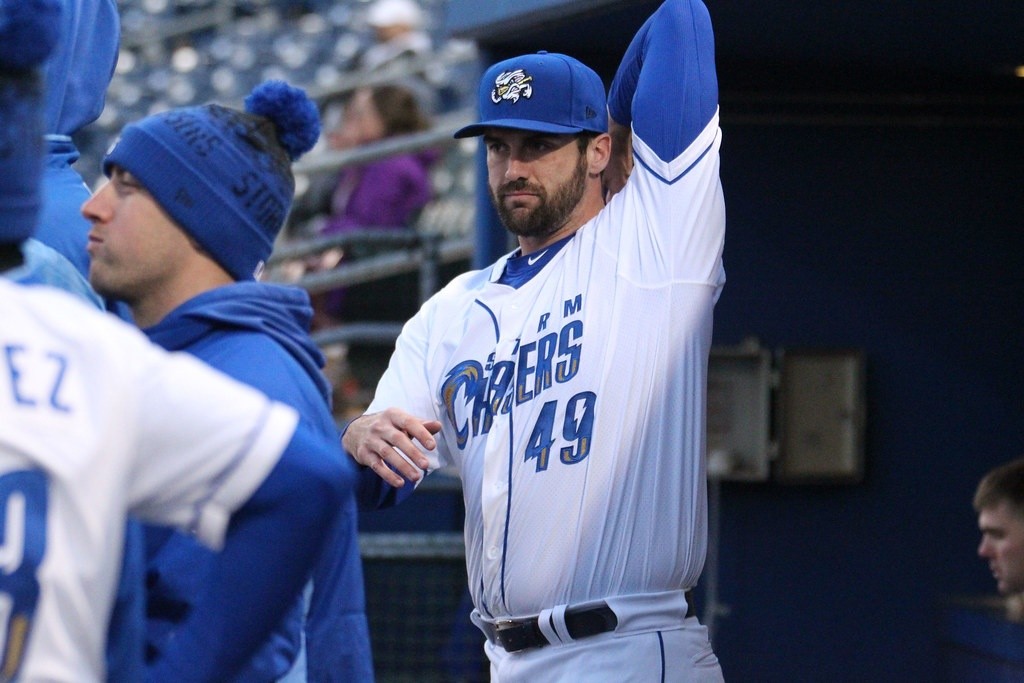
<box><xmin>472</xmin><ymin>590</ymin><xmax>698</xmax><ymax>651</ymax></box>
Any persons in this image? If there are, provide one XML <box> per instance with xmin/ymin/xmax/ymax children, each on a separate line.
<box><xmin>341</xmin><ymin>0</ymin><xmax>727</xmax><ymax>682</ymax></box>
<box><xmin>0</xmin><ymin>2</ymin><xmax>112</xmax><ymax>309</ymax></box>
<box><xmin>81</xmin><ymin>79</ymin><xmax>375</xmax><ymax>683</ymax></box>
<box><xmin>0</xmin><ymin>281</ymin><xmax>360</xmax><ymax>682</ymax></box>
<box><xmin>972</xmin><ymin>458</ymin><xmax>1024</xmax><ymax>624</ymax></box>
<box><xmin>29</xmin><ymin>0</ymin><xmax>151</xmax><ymax>682</ymax></box>
<box><xmin>285</xmin><ymin>0</ymin><xmax>447</xmax><ymax>406</ymax></box>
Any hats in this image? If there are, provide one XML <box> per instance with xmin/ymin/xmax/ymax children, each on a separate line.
<box><xmin>452</xmin><ymin>51</ymin><xmax>609</xmax><ymax>139</ymax></box>
<box><xmin>0</xmin><ymin>0</ymin><xmax>63</xmax><ymax>244</ymax></box>
<box><xmin>100</xmin><ymin>81</ymin><xmax>320</xmax><ymax>279</ymax></box>
